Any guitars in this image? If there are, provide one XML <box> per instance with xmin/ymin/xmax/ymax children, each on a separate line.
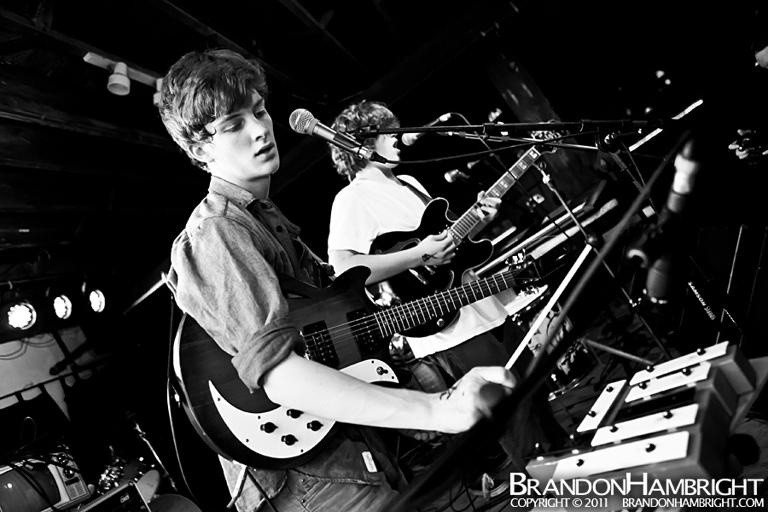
<box><xmin>366</xmin><ymin>117</ymin><xmax>570</xmax><ymax>337</ymax></box>
<box><xmin>172</xmin><ymin>247</ymin><xmax>544</xmax><ymax>469</ymax></box>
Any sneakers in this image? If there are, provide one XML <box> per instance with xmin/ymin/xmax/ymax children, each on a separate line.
<box><xmin>549</xmin><ymin>432</ymin><xmax>587</xmax><ymax>454</ymax></box>
<box><xmin>469</xmin><ymin>477</ymin><xmax>509</xmax><ymax>498</ymax></box>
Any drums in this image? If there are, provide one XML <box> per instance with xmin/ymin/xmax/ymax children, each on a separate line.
<box><xmin>95</xmin><ymin>457</ymin><xmax>160</xmax><ymax>504</ymax></box>
<box><xmin>506</xmin><ymin>286</ymin><xmax>601</xmax><ymax>403</ymax></box>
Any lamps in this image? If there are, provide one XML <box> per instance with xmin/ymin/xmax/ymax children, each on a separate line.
<box><xmin>2</xmin><ymin>295</ymin><xmax>38</xmax><ymax>331</ymax></box>
<box><xmin>107</xmin><ymin>62</ymin><xmax>132</xmax><ymax>98</ymax></box>
<box><xmin>52</xmin><ymin>294</ymin><xmax>73</xmax><ymax>320</ymax></box>
<box><xmin>86</xmin><ymin>287</ymin><xmax>107</xmax><ymax>313</ymax></box>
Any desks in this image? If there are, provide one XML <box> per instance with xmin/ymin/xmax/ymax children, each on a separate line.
<box><xmin>79</xmin><ymin>479</ymin><xmax>136</xmax><ymax>512</ymax></box>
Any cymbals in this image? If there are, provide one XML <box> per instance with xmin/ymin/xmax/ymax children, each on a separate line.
<box><xmin>149</xmin><ymin>494</ymin><xmax>200</xmax><ymax>512</ymax></box>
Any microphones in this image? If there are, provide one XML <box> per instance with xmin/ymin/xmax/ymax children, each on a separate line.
<box><xmin>642</xmin><ymin>151</ymin><xmax>699</xmax><ymax>305</ymax></box>
<box><xmin>400</xmin><ymin>113</ymin><xmax>451</xmax><ymax>145</ymax></box>
<box><xmin>286</xmin><ymin>107</ymin><xmax>387</xmax><ymax>164</ymax></box>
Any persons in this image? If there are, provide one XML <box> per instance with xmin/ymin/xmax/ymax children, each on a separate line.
<box><xmin>158</xmin><ymin>50</ymin><xmax>516</xmax><ymax>512</ymax></box>
<box><xmin>324</xmin><ymin>102</ymin><xmax>575</xmax><ymax>495</ymax></box>
